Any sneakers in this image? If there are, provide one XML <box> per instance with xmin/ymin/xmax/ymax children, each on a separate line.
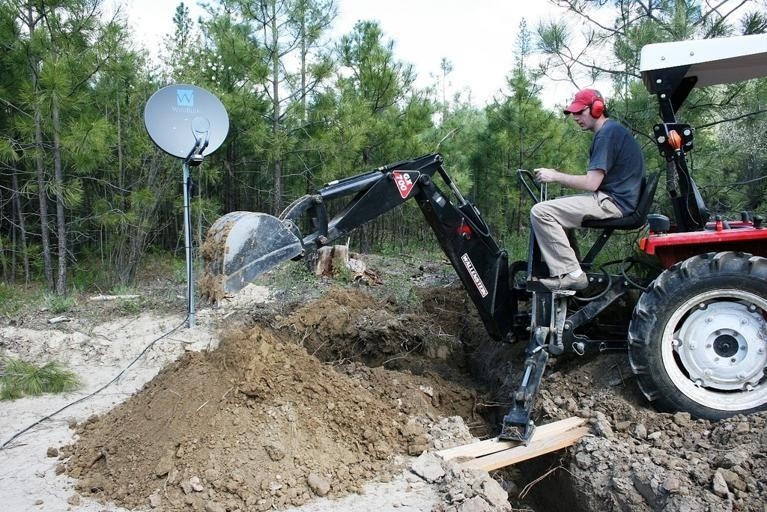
<box><xmin>540</xmin><ymin>272</ymin><xmax>589</xmax><ymax>290</ymax></box>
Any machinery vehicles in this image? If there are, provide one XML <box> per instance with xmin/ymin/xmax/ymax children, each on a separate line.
<box><xmin>201</xmin><ymin>33</ymin><xmax>766</xmax><ymax>444</ymax></box>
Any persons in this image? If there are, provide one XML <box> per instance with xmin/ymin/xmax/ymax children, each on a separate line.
<box><xmin>530</xmin><ymin>90</ymin><xmax>644</xmax><ymax>290</ymax></box>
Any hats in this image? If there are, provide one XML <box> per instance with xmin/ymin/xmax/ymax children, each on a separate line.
<box><xmin>564</xmin><ymin>89</ymin><xmax>604</xmax><ymax>114</ymax></box>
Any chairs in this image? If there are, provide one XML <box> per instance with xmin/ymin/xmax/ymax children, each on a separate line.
<box><xmin>579</xmin><ymin>169</ymin><xmax>659</xmax><ymax>230</ymax></box>
